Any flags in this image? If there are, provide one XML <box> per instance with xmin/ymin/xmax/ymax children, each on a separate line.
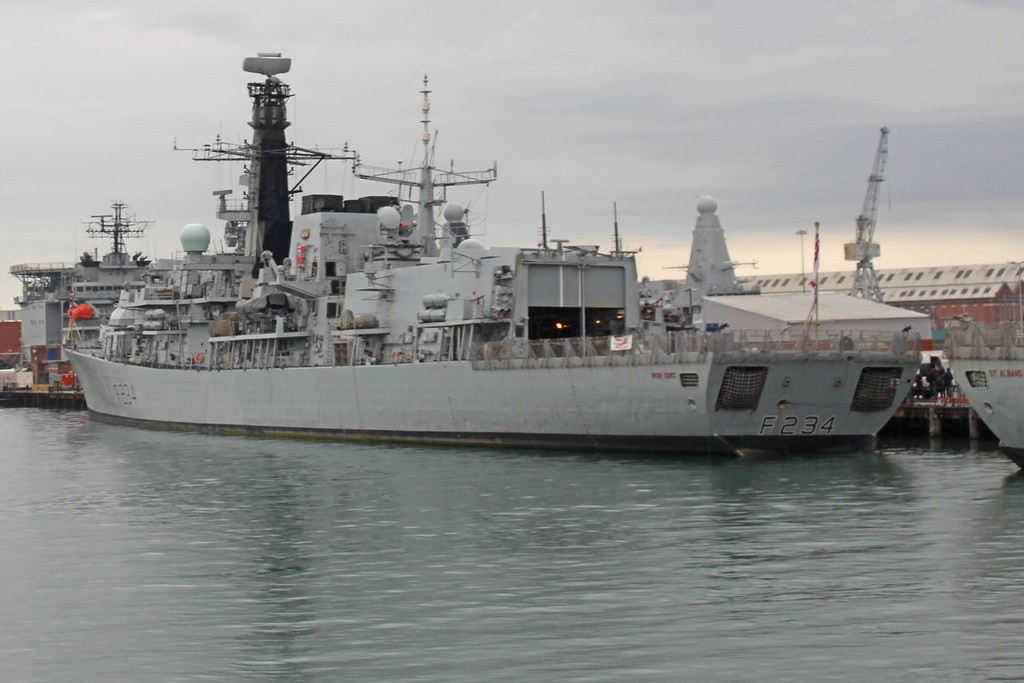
<box><xmin>810</xmin><ymin>232</ymin><xmax>819</xmax><ymax>308</ymax></box>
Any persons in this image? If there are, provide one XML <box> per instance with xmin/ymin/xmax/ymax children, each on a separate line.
<box><xmin>915</xmin><ymin>368</ymin><xmax>953</xmax><ymax>400</ymax></box>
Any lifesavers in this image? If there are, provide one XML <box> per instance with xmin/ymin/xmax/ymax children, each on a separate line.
<box><xmin>391</xmin><ymin>352</ymin><xmax>399</xmax><ymax>363</ymax></box>
<box><xmin>194</xmin><ymin>353</ymin><xmax>204</xmax><ymax>362</ymax></box>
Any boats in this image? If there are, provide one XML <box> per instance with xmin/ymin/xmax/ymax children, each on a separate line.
<box><xmin>60</xmin><ymin>49</ymin><xmax>926</xmax><ymax>452</ymax></box>
<box><xmin>0</xmin><ymin>201</ymin><xmax>182</xmax><ymax>408</ymax></box>
<box><xmin>941</xmin><ymin>258</ymin><xmax>1024</xmax><ymax>470</ymax></box>
